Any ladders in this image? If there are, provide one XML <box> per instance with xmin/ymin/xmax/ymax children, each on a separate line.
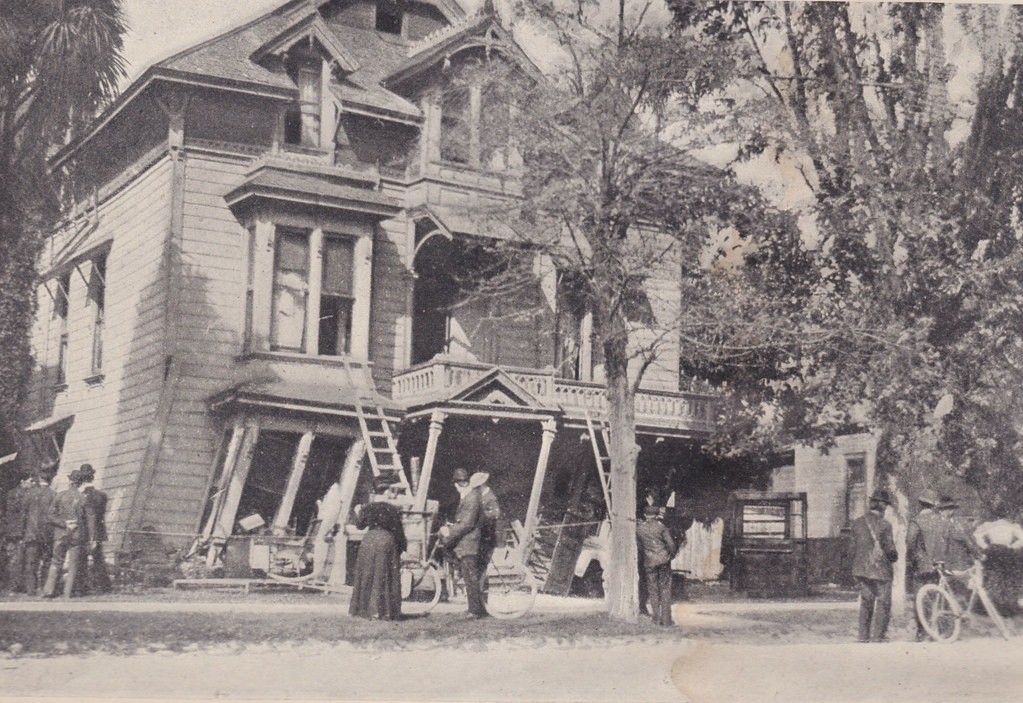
<box><xmin>584</xmin><ymin>410</ymin><xmax>612</xmax><ymax>524</ymax></box>
<box><xmin>342</xmin><ymin>349</ymin><xmax>411</xmax><ymax>497</ymax></box>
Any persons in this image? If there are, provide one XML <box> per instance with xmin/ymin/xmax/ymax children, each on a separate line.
<box><xmin>636</xmin><ymin>506</ymin><xmax>677</xmax><ymax>628</ymax></box>
<box><xmin>436</xmin><ymin>466</ymin><xmax>487</xmax><ymax>620</ymax></box>
<box><xmin>466</xmin><ymin>472</ymin><xmax>501</xmax><ymax>596</ymax></box>
<box><xmin>636</xmin><ymin>486</ymin><xmax>669</xmax><ymax>620</ymax></box>
<box><xmin>905</xmin><ymin>487</ymin><xmax>1023</xmax><ymax>643</ymax></box>
<box><xmin>1</xmin><ymin>462</ymin><xmax>112</xmax><ymax>598</ymax></box>
<box><xmin>851</xmin><ymin>487</ymin><xmax>900</xmax><ymax>643</ymax></box>
<box><xmin>348</xmin><ymin>474</ymin><xmax>410</xmax><ymax>619</ymax></box>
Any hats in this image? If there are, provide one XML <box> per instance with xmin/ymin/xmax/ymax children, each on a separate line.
<box><xmin>917</xmin><ymin>490</ymin><xmax>936</xmax><ymax>506</ymax></box>
<box><xmin>869</xmin><ymin>490</ymin><xmax>891</xmax><ymax>505</ymax></box>
<box><xmin>470</xmin><ymin>472</ymin><xmax>490</xmax><ymax>489</ymax></box>
<box><xmin>452</xmin><ymin>468</ymin><xmax>468</xmax><ymax>481</ymax></box>
<box><xmin>937</xmin><ymin>497</ymin><xmax>960</xmax><ymax>509</ymax></box>
<box><xmin>79</xmin><ymin>464</ymin><xmax>95</xmax><ymax>476</ymax></box>
<box><xmin>67</xmin><ymin>470</ymin><xmax>84</xmax><ymax>484</ymax></box>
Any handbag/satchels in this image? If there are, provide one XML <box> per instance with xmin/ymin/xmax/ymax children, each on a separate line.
<box><xmin>866</xmin><ymin>541</ymin><xmax>892</xmax><ymax>579</ymax></box>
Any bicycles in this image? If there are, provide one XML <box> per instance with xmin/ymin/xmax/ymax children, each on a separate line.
<box><xmin>915</xmin><ymin>544</ymin><xmax>1022</xmax><ymax>645</ymax></box>
<box><xmin>371</xmin><ymin>521</ymin><xmax>540</xmax><ymax>621</ymax></box>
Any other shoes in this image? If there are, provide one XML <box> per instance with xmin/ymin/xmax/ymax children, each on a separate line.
<box><xmin>660</xmin><ymin>620</ymin><xmax>678</xmax><ymax>627</ymax></box>
<box><xmin>854</xmin><ymin>636</ymin><xmax>869</xmax><ymax>642</ymax></box>
<box><xmin>457</xmin><ymin>612</ymin><xmax>479</xmax><ymax>621</ymax></box>
<box><xmin>873</xmin><ymin>636</ymin><xmax>889</xmax><ymax>642</ymax></box>
